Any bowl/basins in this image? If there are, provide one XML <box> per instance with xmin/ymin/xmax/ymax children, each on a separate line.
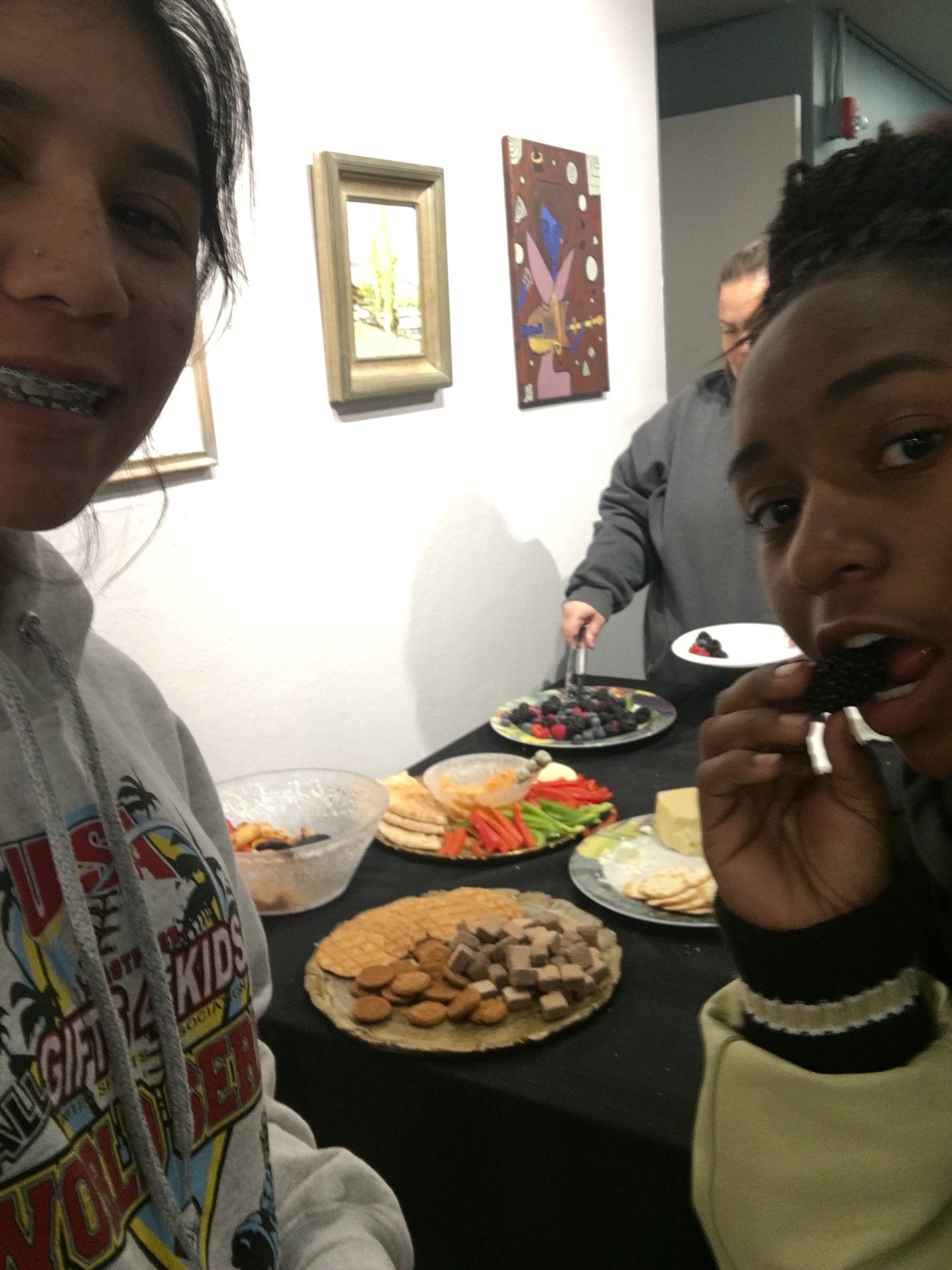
<box><xmin>214</xmin><ymin>766</ymin><xmax>388</xmax><ymax>917</ymax></box>
<box><xmin>424</xmin><ymin>751</ymin><xmax>541</xmax><ymax>810</ymax></box>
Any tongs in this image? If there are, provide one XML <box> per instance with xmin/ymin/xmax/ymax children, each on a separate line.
<box><xmin>563</xmin><ymin>623</ymin><xmax>587</xmax><ymax>692</ymax></box>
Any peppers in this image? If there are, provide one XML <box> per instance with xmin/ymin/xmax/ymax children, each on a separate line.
<box><xmin>443</xmin><ymin>774</ymin><xmax>614</xmax><ymax>856</ymax></box>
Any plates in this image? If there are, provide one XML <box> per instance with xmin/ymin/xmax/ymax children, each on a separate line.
<box><xmin>487</xmin><ymin>685</ymin><xmax>679</xmax><ymax>750</ymax></box>
<box><xmin>375</xmin><ymin>773</ymin><xmax>610</xmax><ymax>859</ymax></box>
<box><xmin>304</xmin><ymin>886</ymin><xmax>622</xmax><ymax>1053</ymax></box>
<box><xmin>670</xmin><ymin>620</ymin><xmax>802</xmax><ymax>670</ymax></box>
<box><xmin>568</xmin><ymin>811</ymin><xmax>720</xmax><ymax>929</ymax></box>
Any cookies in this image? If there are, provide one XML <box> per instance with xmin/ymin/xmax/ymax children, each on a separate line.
<box><xmin>624</xmin><ymin>865</ymin><xmax>717</xmax><ymax>914</ymax></box>
<box><xmin>317</xmin><ymin>886</ymin><xmax>521</xmax><ymax>1027</ymax></box>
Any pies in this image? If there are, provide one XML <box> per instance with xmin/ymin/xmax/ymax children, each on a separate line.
<box><xmin>377</xmin><ymin>772</ymin><xmax>447</xmax><ymax>852</ymax></box>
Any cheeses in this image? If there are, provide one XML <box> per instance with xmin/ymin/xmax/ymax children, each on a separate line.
<box><xmin>655</xmin><ymin>786</ymin><xmax>704</xmax><ymax>856</ymax></box>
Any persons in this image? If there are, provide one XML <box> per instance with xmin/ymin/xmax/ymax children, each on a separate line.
<box><xmin>564</xmin><ymin>236</ymin><xmax>783</xmax><ymax>690</ymax></box>
<box><xmin>0</xmin><ymin>0</ymin><xmax>413</xmax><ymax>1270</ymax></box>
<box><xmin>690</xmin><ymin>110</ymin><xmax>952</xmax><ymax>1270</ymax></box>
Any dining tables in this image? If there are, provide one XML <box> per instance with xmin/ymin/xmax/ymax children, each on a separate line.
<box><xmin>238</xmin><ymin>672</ymin><xmax>928</xmax><ymax>1270</ymax></box>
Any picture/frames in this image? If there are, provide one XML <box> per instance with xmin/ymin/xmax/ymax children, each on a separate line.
<box><xmin>96</xmin><ymin>298</ymin><xmax>219</xmax><ymax>495</ymax></box>
<box><xmin>312</xmin><ymin>150</ymin><xmax>453</xmax><ymax>404</ymax></box>
